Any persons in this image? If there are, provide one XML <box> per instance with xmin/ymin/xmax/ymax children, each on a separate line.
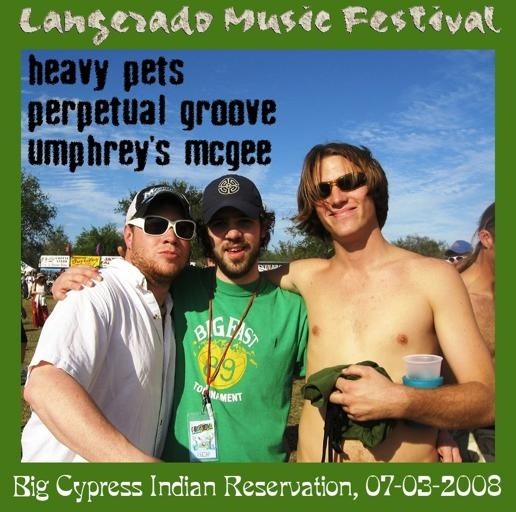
<box><xmin>52</xmin><ymin>142</ymin><xmax>496</xmax><ymax>464</ymax></box>
<box><xmin>446</xmin><ymin>202</ymin><xmax>496</xmax><ymax>463</ymax></box>
<box><xmin>19</xmin><ymin>184</ymin><xmax>198</xmax><ymax>465</ymax></box>
<box><xmin>31</xmin><ymin>272</ymin><xmax>49</xmax><ymax>328</ymax></box>
<box><xmin>156</xmin><ymin>175</ymin><xmax>465</xmax><ymax>463</ymax></box>
<box><xmin>446</xmin><ymin>240</ymin><xmax>474</xmax><ymax>269</ymax></box>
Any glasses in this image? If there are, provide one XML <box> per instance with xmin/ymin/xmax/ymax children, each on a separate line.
<box><xmin>127</xmin><ymin>216</ymin><xmax>197</xmax><ymax>240</ymax></box>
<box><xmin>305</xmin><ymin>171</ymin><xmax>367</xmax><ymax>201</ymax></box>
<box><xmin>448</xmin><ymin>256</ymin><xmax>464</xmax><ymax>264</ymax></box>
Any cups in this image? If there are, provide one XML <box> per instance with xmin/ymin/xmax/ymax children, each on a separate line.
<box><xmin>402</xmin><ymin>354</ymin><xmax>443</xmax><ymax>427</ymax></box>
<box><xmin>41</xmin><ymin>295</ymin><xmax>47</xmax><ymax>307</ymax></box>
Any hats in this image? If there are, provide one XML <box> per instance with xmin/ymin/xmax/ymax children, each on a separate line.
<box><xmin>126</xmin><ymin>184</ymin><xmax>191</xmax><ymax>220</ymax></box>
<box><xmin>34</xmin><ymin>273</ymin><xmax>46</xmax><ymax>280</ymax></box>
<box><xmin>202</xmin><ymin>174</ymin><xmax>262</xmax><ymax>224</ymax></box>
<box><xmin>445</xmin><ymin>240</ymin><xmax>475</xmax><ymax>255</ymax></box>
